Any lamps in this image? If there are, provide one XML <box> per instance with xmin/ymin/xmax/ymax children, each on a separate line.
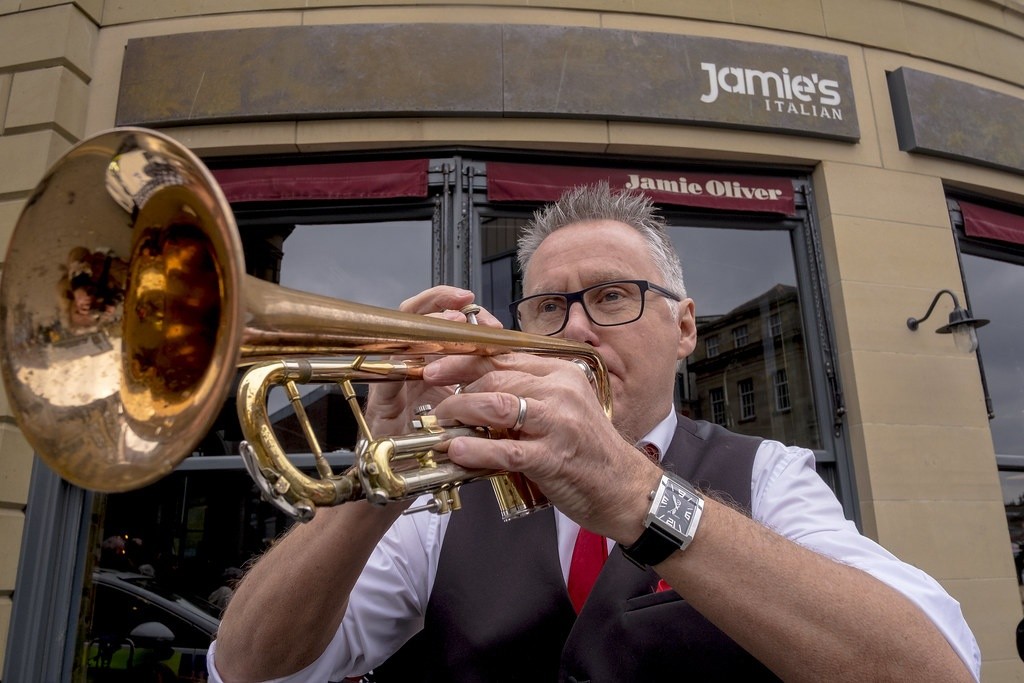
<box><xmin>907</xmin><ymin>289</ymin><xmax>989</xmax><ymax>355</ymax></box>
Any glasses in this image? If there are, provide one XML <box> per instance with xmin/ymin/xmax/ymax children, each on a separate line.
<box><xmin>507</xmin><ymin>279</ymin><xmax>680</xmax><ymax>337</ymax></box>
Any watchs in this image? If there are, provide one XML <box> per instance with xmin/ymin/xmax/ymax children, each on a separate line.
<box><xmin>619</xmin><ymin>469</ymin><xmax>706</xmax><ymax>571</ymax></box>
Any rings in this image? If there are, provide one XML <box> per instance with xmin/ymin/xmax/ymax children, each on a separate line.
<box><xmin>512</xmin><ymin>396</ymin><xmax>527</xmax><ymax>431</ymax></box>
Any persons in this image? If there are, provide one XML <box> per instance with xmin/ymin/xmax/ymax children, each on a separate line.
<box><xmin>206</xmin><ymin>182</ymin><xmax>983</xmax><ymax>683</ymax></box>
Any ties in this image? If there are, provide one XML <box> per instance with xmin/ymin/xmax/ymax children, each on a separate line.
<box><xmin>566</xmin><ymin>444</ymin><xmax>658</xmax><ymax>615</ymax></box>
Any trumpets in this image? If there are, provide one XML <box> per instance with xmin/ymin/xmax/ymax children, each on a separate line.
<box><xmin>0</xmin><ymin>124</ymin><xmax>615</xmax><ymax>524</ymax></box>
